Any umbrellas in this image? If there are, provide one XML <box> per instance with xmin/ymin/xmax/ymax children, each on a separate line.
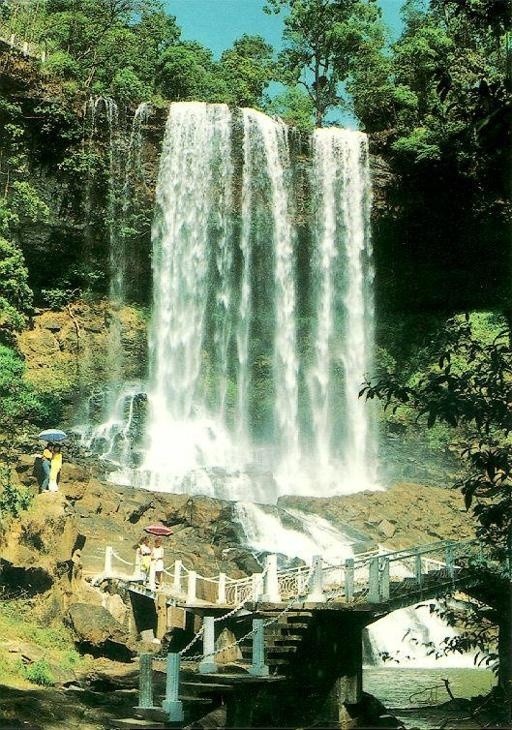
<box><xmin>38</xmin><ymin>429</ymin><xmax>68</xmax><ymax>442</ymax></box>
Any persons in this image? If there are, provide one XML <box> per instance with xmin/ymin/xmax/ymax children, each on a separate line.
<box><xmin>134</xmin><ymin>537</ymin><xmax>164</xmax><ymax>584</ymax></box>
<box><xmin>47</xmin><ymin>444</ymin><xmax>62</xmax><ymax>493</ymax></box>
<box><xmin>41</xmin><ymin>442</ymin><xmax>53</xmax><ymax>493</ymax></box>
